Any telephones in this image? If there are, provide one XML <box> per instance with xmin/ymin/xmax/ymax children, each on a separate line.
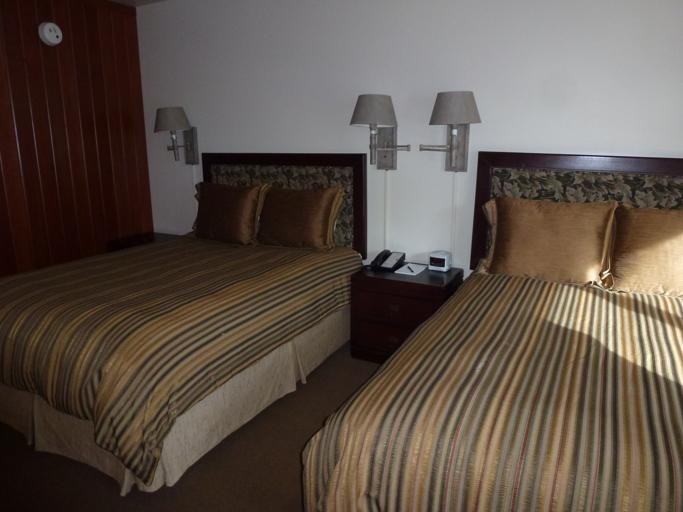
<box><xmin>371</xmin><ymin>250</ymin><xmax>405</xmax><ymax>271</ymax></box>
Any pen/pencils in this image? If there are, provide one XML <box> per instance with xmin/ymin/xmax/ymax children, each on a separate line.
<box><xmin>408</xmin><ymin>266</ymin><xmax>413</xmax><ymax>272</ymax></box>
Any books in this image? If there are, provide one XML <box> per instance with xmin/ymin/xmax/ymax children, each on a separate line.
<box><xmin>394</xmin><ymin>263</ymin><xmax>426</xmax><ymax>276</ymax></box>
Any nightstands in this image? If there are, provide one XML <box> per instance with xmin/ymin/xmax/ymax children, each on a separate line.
<box><xmin>349</xmin><ymin>265</ymin><xmax>464</xmax><ymax>364</ymax></box>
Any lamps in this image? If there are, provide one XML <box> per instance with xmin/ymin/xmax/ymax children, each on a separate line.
<box><xmin>154</xmin><ymin>107</ymin><xmax>200</xmax><ymax>165</ymax></box>
<box><xmin>351</xmin><ymin>91</ymin><xmax>482</xmax><ymax>171</ymax></box>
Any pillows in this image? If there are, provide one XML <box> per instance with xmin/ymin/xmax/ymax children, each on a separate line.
<box><xmin>191</xmin><ymin>181</ymin><xmax>346</xmax><ymax>254</ymax></box>
<box><xmin>479</xmin><ymin>196</ymin><xmax>683</xmax><ymax>299</ymax></box>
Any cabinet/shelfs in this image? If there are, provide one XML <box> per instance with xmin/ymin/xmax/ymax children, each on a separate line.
<box><xmin>0</xmin><ymin>0</ymin><xmax>156</xmax><ymax>277</ymax></box>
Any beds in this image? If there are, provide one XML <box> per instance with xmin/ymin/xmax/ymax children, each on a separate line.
<box><xmin>305</xmin><ymin>151</ymin><xmax>683</xmax><ymax>512</ymax></box>
<box><xmin>1</xmin><ymin>152</ymin><xmax>367</xmax><ymax>490</ymax></box>
